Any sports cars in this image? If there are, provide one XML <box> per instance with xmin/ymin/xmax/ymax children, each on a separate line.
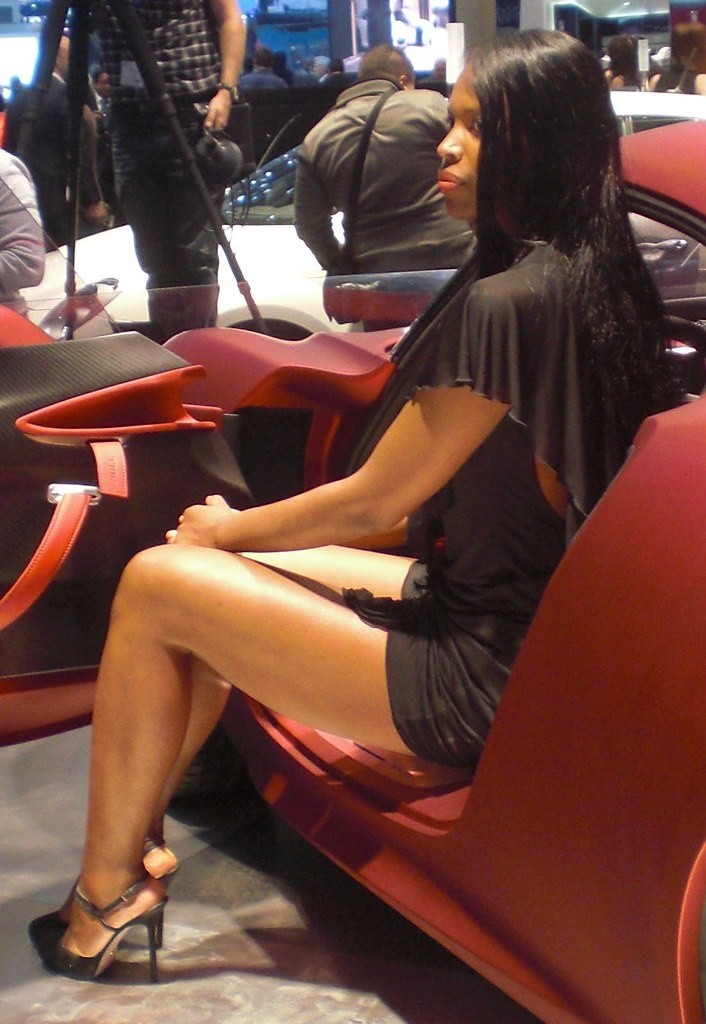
<box><xmin>1</xmin><ymin>120</ymin><xmax>706</xmax><ymax>1024</ymax></box>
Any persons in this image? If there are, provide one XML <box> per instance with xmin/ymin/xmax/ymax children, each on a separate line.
<box><xmin>239</xmin><ymin>26</ymin><xmax>345</xmax><ymax>92</ymax></box>
<box><xmin>84</xmin><ymin>0</ymin><xmax>247</xmax><ymax>345</ymax></box>
<box><xmin>0</xmin><ymin>31</ymin><xmax>124</xmax><ymax>246</ymax></box>
<box><xmin>604</xmin><ymin>22</ymin><xmax>706</xmax><ymax>95</ymax></box>
<box><xmin>294</xmin><ymin>43</ymin><xmax>479</xmax><ymax>333</ymax></box>
<box><xmin>28</xmin><ymin>29</ymin><xmax>685</xmax><ymax>982</ymax></box>
<box><xmin>417</xmin><ymin>56</ymin><xmax>446</xmax><ymax>83</ymax></box>
<box><xmin>0</xmin><ymin>148</ymin><xmax>46</xmax><ymax>316</ymax></box>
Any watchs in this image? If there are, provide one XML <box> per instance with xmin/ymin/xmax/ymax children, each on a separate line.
<box><xmin>218</xmin><ymin>83</ymin><xmax>240</xmax><ymax>102</ymax></box>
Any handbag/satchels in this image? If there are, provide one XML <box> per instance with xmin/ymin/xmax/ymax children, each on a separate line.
<box><xmin>325</xmin><ymin>244</ymin><xmax>361</xmax><ymax>326</ymax></box>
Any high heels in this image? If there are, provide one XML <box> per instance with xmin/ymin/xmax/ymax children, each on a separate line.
<box><xmin>26</xmin><ymin>839</ymin><xmax>181</xmax><ymax>949</ymax></box>
<box><xmin>35</xmin><ymin>870</ymin><xmax>169</xmax><ymax>985</ymax></box>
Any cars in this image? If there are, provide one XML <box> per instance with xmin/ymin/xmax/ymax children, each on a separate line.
<box><xmin>20</xmin><ymin>91</ymin><xmax>706</xmax><ymax>344</ymax></box>
